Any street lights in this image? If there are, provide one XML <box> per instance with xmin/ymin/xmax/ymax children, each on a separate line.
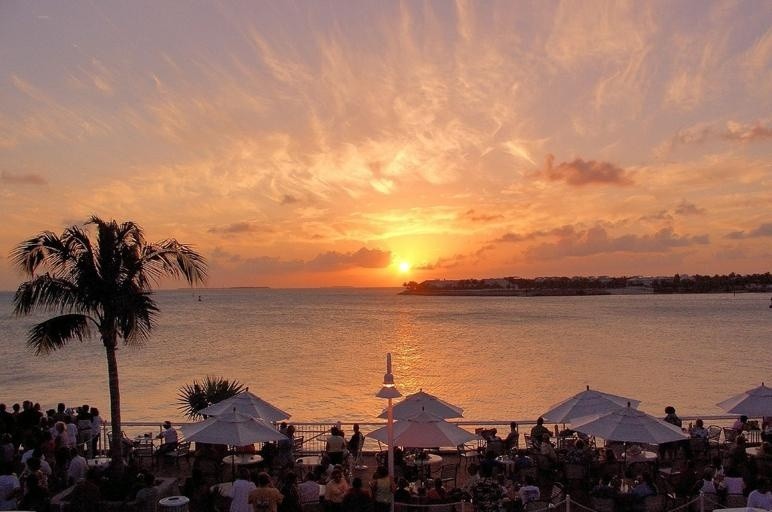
<box><xmin>375</xmin><ymin>352</ymin><xmax>402</xmax><ymax>482</ymax></box>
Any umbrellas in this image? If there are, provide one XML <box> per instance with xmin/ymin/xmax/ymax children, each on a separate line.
<box><xmin>540</xmin><ymin>384</ymin><xmax>642</xmax><ymax>422</ymax></box>
<box><xmin>716</xmin><ymin>382</ymin><xmax>770</xmax><ymax>417</ymax></box>
<box><xmin>376</xmin><ymin>389</ymin><xmax>465</xmax><ymax>421</ymax></box>
<box><xmin>197</xmin><ymin>388</ymin><xmax>293</xmax><ymax>420</ymax></box>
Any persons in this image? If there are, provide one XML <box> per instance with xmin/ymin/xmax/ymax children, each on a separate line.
<box><xmin>2</xmin><ymin>399</ymin><xmax>772</xmax><ymax>512</ymax></box>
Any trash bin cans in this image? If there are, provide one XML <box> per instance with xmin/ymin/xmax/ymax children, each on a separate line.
<box><xmin>158</xmin><ymin>496</ymin><xmax>190</xmax><ymax>511</ymax></box>
